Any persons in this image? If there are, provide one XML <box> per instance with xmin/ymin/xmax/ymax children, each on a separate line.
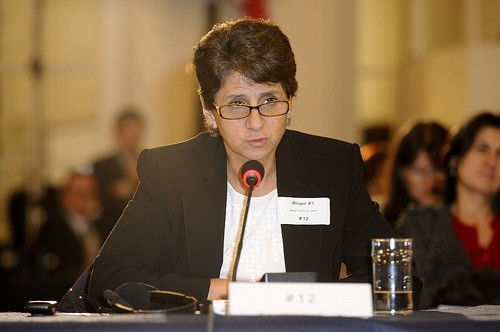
<box><xmin>28</xmin><ymin>111</ymin><xmax>143</xmax><ymax>285</ymax></box>
<box><xmin>87</xmin><ymin>18</ymin><xmax>422</xmax><ymax>312</ymax></box>
<box><xmin>381</xmin><ymin>110</ymin><xmax>497</xmax><ymax>307</ymax></box>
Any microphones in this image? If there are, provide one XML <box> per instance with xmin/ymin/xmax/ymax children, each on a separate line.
<box><xmin>232</xmin><ymin>160</ymin><xmax>265</xmax><ymax>281</ymax></box>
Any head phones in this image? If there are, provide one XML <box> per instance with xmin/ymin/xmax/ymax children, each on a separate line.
<box><xmin>103</xmin><ymin>283</ymin><xmax>198</xmax><ymax>313</ymax></box>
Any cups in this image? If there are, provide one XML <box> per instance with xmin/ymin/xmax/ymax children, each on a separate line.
<box><xmin>370</xmin><ymin>237</ymin><xmax>414</xmax><ymax>317</ymax></box>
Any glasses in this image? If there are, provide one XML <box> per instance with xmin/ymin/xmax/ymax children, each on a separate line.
<box><xmin>210</xmin><ymin>93</ymin><xmax>291</xmax><ymax>120</ymax></box>
